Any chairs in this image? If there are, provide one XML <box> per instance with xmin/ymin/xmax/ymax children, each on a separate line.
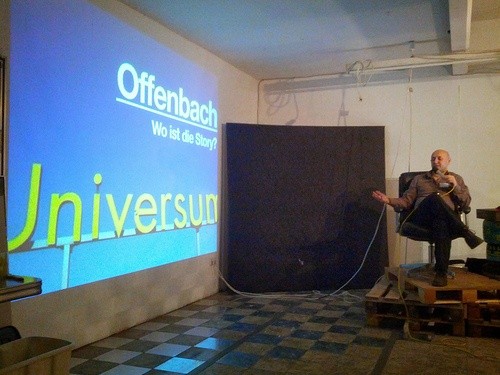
<box><xmin>395</xmin><ymin>172</ymin><xmax>471</xmax><ymax>280</ymax></box>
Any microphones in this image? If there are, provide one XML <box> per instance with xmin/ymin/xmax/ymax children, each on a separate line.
<box><xmin>432</xmin><ymin>167</ymin><xmax>453</xmax><ymax>187</ymax></box>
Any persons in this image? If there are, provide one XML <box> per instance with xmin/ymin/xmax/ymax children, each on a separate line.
<box><xmin>372</xmin><ymin>148</ymin><xmax>484</xmax><ymax>288</ymax></box>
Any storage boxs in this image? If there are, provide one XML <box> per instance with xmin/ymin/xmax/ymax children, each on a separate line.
<box><xmin>0</xmin><ymin>335</ymin><xmax>73</xmax><ymax>375</ymax></box>
